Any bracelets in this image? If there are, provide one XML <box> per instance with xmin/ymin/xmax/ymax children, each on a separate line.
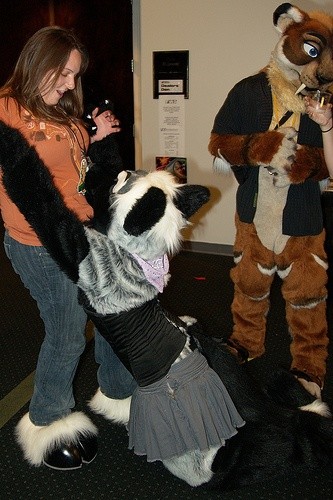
<box><xmin>319</xmin><ymin>127</ymin><xmax>333</xmax><ymax>133</ymax></box>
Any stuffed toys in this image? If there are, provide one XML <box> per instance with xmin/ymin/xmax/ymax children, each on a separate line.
<box><xmin>208</xmin><ymin>1</ymin><xmax>332</xmax><ymax>401</ymax></box>
<box><xmin>1</xmin><ymin>120</ymin><xmax>332</xmax><ymax>491</ymax></box>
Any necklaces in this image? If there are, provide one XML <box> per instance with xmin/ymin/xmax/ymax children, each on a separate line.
<box><xmin>62</xmin><ymin>117</ymin><xmax>89</xmax><ymax>197</ymax></box>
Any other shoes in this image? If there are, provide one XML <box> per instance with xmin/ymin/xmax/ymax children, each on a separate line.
<box><xmin>17</xmin><ymin>411</ymin><xmax>99</xmax><ymax>470</ymax></box>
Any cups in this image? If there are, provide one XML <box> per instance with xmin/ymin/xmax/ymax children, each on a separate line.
<box><xmin>308</xmin><ymin>90</ymin><xmax>332</xmax><ymax>117</ymax></box>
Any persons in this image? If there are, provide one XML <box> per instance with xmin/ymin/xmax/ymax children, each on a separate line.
<box><xmin>0</xmin><ymin>25</ymin><xmax>135</xmax><ymax>459</ymax></box>
<box><xmin>304</xmin><ymin>96</ymin><xmax>333</xmax><ymax>180</ymax></box>
